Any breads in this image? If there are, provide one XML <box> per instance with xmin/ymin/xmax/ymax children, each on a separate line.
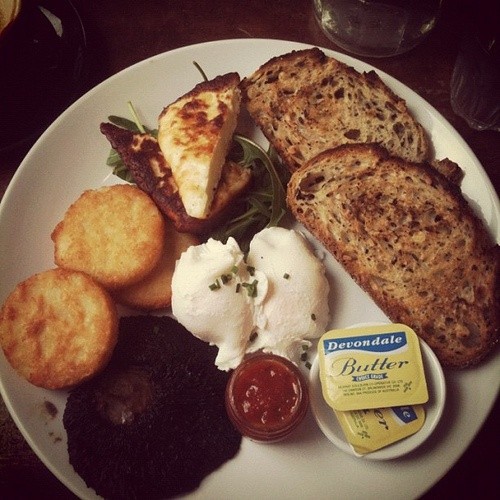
<box><xmin>100</xmin><ymin>48</ymin><xmax>499</xmax><ymax>373</ymax></box>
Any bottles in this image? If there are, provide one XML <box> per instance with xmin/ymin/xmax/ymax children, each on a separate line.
<box><xmin>450</xmin><ymin>16</ymin><xmax>500</xmax><ymax>132</ymax></box>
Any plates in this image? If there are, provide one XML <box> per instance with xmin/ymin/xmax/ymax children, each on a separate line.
<box><xmin>309</xmin><ymin>322</ymin><xmax>448</xmax><ymax>461</ymax></box>
<box><xmin>0</xmin><ymin>40</ymin><xmax>500</xmax><ymax>500</ymax></box>
<box><xmin>1</xmin><ymin>0</ymin><xmax>88</xmax><ymax>166</ymax></box>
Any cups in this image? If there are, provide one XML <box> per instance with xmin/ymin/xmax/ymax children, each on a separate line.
<box><xmin>314</xmin><ymin>0</ymin><xmax>443</xmax><ymax>58</ymax></box>
